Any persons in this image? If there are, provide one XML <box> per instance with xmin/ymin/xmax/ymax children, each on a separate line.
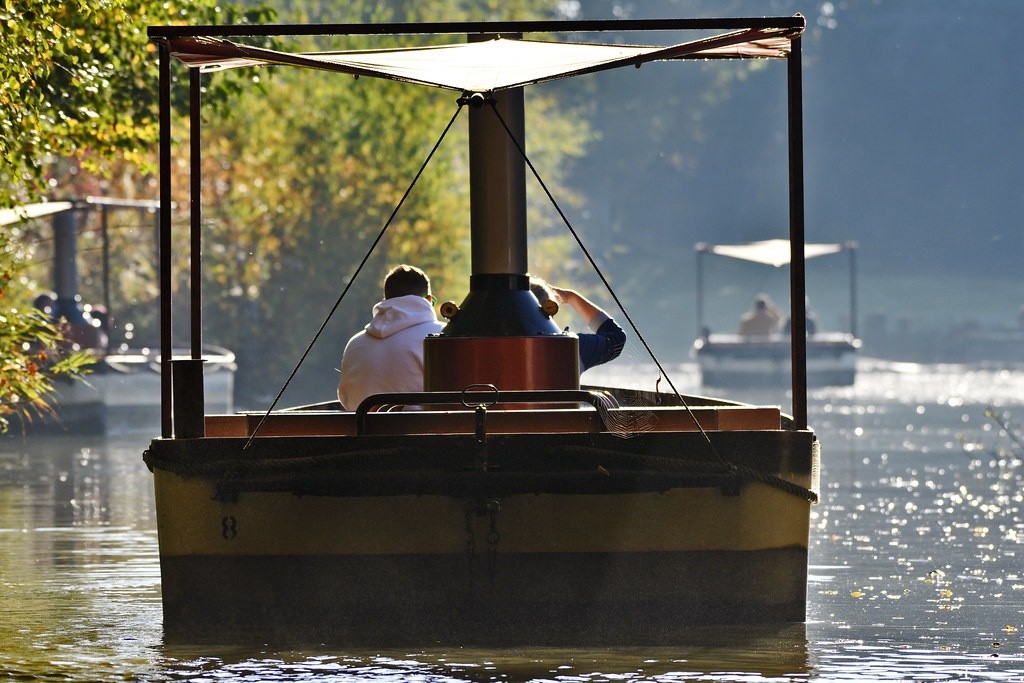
<box><xmin>337</xmin><ymin>265</ymin><xmax>449</xmax><ymax>412</ymax></box>
<box><xmin>32</xmin><ymin>294</ymin><xmax>114</xmax><ymax>360</ymax></box>
<box><xmin>530</xmin><ymin>278</ymin><xmax>627</xmax><ymax>377</ymax></box>
<box><xmin>785</xmin><ymin>306</ymin><xmax>815</xmax><ymax>335</ymax></box>
<box><xmin>742</xmin><ymin>301</ymin><xmax>780</xmax><ymax>336</ymax></box>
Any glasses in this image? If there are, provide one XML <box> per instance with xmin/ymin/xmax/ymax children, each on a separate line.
<box><xmin>422</xmin><ymin>294</ymin><xmax>437</xmax><ymax>306</ymax></box>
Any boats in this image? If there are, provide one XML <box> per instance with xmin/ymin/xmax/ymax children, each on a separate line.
<box><xmin>691</xmin><ymin>237</ymin><xmax>862</xmax><ymax>391</ymax></box>
<box><xmin>0</xmin><ymin>198</ymin><xmax>239</xmax><ymax>433</ymax></box>
<box><xmin>142</xmin><ymin>13</ymin><xmax>823</xmax><ymax>655</ymax></box>
